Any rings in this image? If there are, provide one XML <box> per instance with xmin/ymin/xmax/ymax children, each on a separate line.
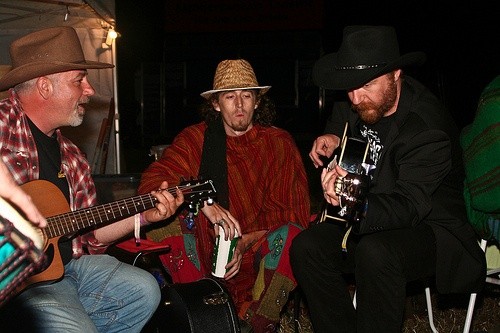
<box><xmin>219</xmin><ymin>220</ymin><xmax>226</xmax><ymax>224</ymax></box>
<box><xmin>213</xmin><ymin>223</ymin><xmax>219</xmax><ymax>226</ymax></box>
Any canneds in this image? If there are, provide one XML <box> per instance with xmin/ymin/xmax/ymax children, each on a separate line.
<box><xmin>211</xmin><ymin>225</ymin><xmax>238</xmax><ymax>279</ymax></box>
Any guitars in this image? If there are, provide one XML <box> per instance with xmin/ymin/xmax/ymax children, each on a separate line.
<box><xmin>317</xmin><ymin>120</ymin><xmax>369</xmax><ymax>221</ymax></box>
<box><xmin>17</xmin><ymin>176</ymin><xmax>216</xmax><ymax>293</ymax></box>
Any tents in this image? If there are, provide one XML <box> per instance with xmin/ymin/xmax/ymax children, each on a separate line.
<box><xmin>0</xmin><ymin>0</ymin><xmax>120</xmax><ymax>175</ymax></box>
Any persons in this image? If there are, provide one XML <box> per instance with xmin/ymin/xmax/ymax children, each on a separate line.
<box><xmin>139</xmin><ymin>60</ymin><xmax>310</xmax><ymax>333</ymax></box>
<box><xmin>288</xmin><ymin>28</ymin><xmax>486</xmax><ymax>333</ymax></box>
<box><xmin>0</xmin><ymin>26</ymin><xmax>184</xmax><ymax>333</ymax></box>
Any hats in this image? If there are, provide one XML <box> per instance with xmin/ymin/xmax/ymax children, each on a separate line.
<box><xmin>311</xmin><ymin>23</ymin><xmax>401</xmax><ymax>90</ymax></box>
<box><xmin>0</xmin><ymin>27</ymin><xmax>115</xmax><ymax>92</ymax></box>
<box><xmin>200</xmin><ymin>59</ymin><xmax>272</xmax><ymax>100</ymax></box>
